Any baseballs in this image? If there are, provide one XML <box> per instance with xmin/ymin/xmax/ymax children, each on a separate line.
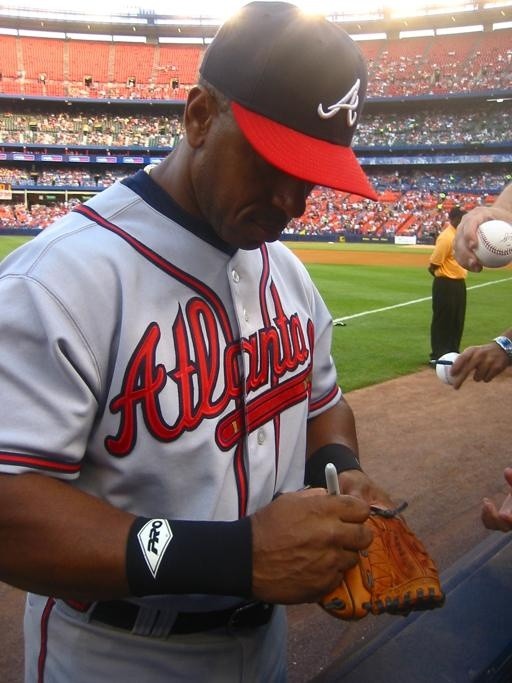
<box><xmin>472</xmin><ymin>220</ymin><xmax>512</xmax><ymax>267</ymax></box>
<box><xmin>436</xmin><ymin>352</ymin><xmax>463</xmax><ymax>383</ymax></box>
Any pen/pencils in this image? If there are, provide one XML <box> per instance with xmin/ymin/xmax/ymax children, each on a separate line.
<box><xmin>430</xmin><ymin>360</ymin><xmax>453</xmax><ymax>365</ymax></box>
<box><xmin>325</xmin><ymin>463</ymin><xmax>340</xmax><ymax>496</ymax></box>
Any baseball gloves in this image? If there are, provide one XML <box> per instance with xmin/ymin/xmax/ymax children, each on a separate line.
<box><xmin>271</xmin><ymin>483</ymin><xmax>447</xmax><ymax>621</ymax></box>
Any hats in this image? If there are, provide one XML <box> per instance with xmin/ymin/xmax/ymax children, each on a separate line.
<box><xmin>447</xmin><ymin>205</ymin><xmax>469</xmax><ymax>219</ymax></box>
<box><xmin>196</xmin><ymin>0</ymin><xmax>383</xmax><ymax>205</ymax></box>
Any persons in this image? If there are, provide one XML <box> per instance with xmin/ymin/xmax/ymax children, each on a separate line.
<box><xmin>1</xmin><ymin>4</ymin><xmax>409</xmax><ymax>681</ymax></box>
<box><xmin>481</xmin><ymin>467</ymin><xmax>512</xmax><ymax>533</ymax></box>
<box><xmin>0</xmin><ymin>64</ymin><xmax>185</xmax><ymax>229</ymax></box>
<box><xmin>278</xmin><ymin>50</ymin><xmax>512</xmax><ymax>238</ymax></box>
<box><xmin>427</xmin><ymin>178</ymin><xmax>511</xmax><ymax>392</ymax></box>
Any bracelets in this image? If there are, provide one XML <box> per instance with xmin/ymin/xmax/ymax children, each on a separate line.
<box><xmin>305</xmin><ymin>442</ymin><xmax>367</xmax><ymax>491</ymax></box>
<box><xmin>124</xmin><ymin>511</ymin><xmax>256</xmax><ymax>603</ymax></box>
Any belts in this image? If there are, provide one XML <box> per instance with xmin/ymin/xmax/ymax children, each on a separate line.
<box><xmin>61</xmin><ymin>598</ymin><xmax>282</xmax><ymax>639</ymax></box>
